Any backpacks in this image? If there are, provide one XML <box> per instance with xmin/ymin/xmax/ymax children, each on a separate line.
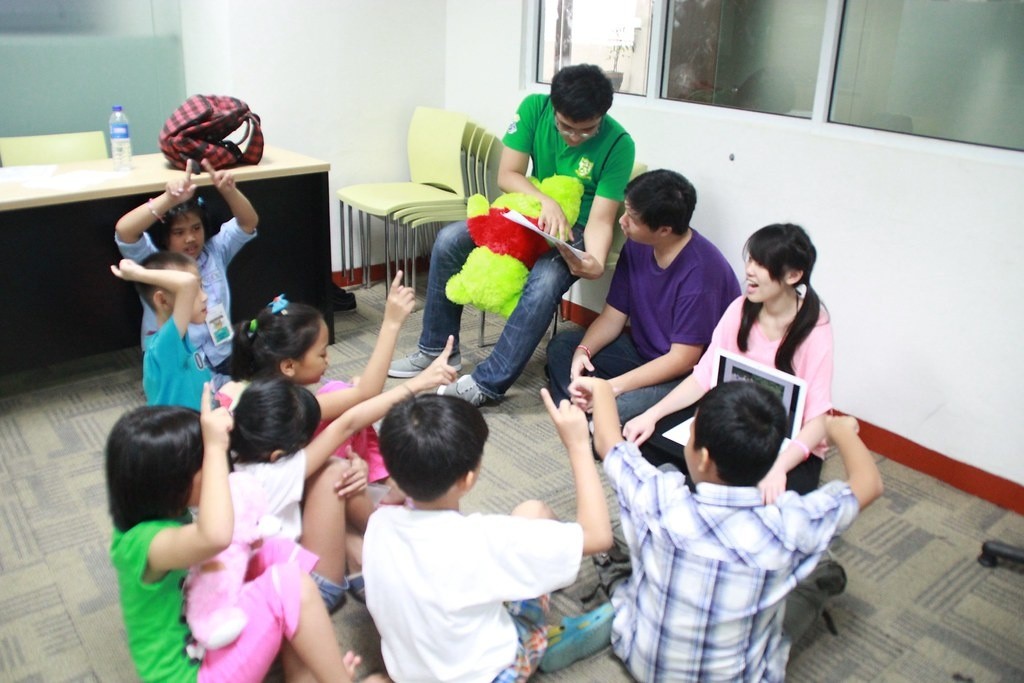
<box><xmin>157</xmin><ymin>94</ymin><xmax>264</xmax><ymax>175</ymax></box>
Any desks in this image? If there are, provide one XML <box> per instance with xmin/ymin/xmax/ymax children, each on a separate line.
<box><xmin>0</xmin><ymin>145</ymin><xmax>335</xmax><ymax>379</ymax></box>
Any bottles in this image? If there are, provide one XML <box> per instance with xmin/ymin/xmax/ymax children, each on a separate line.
<box><xmin>108</xmin><ymin>106</ymin><xmax>132</xmax><ymax>168</ymax></box>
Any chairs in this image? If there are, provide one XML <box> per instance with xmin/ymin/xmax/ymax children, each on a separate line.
<box><xmin>0</xmin><ymin>131</ymin><xmax>108</xmax><ymax>168</ymax></box>
<box><xmin>339</xmin><ymin>106</ymin><xmax>496</xmax><ymax>314</ymax></box>
<box><xmin>478</xmin><ymin>163</ymin><xmax>646</xmax><ymax>349</ymax></box>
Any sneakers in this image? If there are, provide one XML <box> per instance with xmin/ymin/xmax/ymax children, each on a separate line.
<box><xmin>387</xmin><ymin>350</ymin><xmax>462</xmax><ymax>378</ymax></box>
<box><xmin>437</xmin><ymin>374</ymin><xmax>491</xmax><ymax>407</ymax></box>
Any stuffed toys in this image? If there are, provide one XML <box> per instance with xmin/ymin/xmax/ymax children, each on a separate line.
<box><xmin>445</xmin><ymin>174</ymin><xmax>582</xmax><ymax>319</ymax></box>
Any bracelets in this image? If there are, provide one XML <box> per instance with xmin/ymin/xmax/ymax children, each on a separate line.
<box><xmin>147</xmin><ymin>198</ymin><xmax>164</xmax><ymax>223</ymax></box>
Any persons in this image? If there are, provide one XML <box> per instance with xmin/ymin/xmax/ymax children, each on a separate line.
<box><xmin>385</xmin><ymin>63</ymin><xmax>634</xmax><ymax>404</ymax></box>
<box><xmin>620</xmin><ymin>225</ymin><xmax>831</xmax><ymax>500</ymax></box>
<box><xmin>113</xmin><ymin>156</ymin><xmax>257</xmax><ymax>374</ymax></box>
<box><xmin>545</xmin><ymin>166</ymin><xmax>741</xmax><ymax>435</ymax></box>
<box><xmin>111</xmin><ymin>251</ymin><xmax>214</xmax><ymax>413</ymax></box>
<box><xmin>224</xmin><ymin>333</ymin><xmax>458</xmax><ymax>619</ymax></box>
<box><xmin>567</xmin><ymin>372</ymin><xmax>885</xmax><ymax>683</ymax></box>
<box><xmin>364</xmin><ymin>389</ymin><xmax>614</xmax><ymax>683</ymax></box>
<box><xmin>221</xmin><ymin>267</ymin><xmax>416</xmax><ymax>424</ymax></box>
<box><xmin>103</xmin><ymin>380</ymin><xmax>390</xmax><ymax>682</ymax></box>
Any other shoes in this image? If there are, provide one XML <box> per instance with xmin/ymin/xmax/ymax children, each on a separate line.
<box><xmin>331</xmin><ymin>281</ymin><xmax>357</xmax><ymax>315</ymax></box>
<box><xmin>539</xmin><ymin>601</ymin><xmax>615</xmax><ymax>673</ymax></box>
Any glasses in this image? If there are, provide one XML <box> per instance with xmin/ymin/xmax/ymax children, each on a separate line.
<box><xmin>557</xmin><ymin>121</ymin><xmax>599</xmax><ymax>138</ymax></box>
